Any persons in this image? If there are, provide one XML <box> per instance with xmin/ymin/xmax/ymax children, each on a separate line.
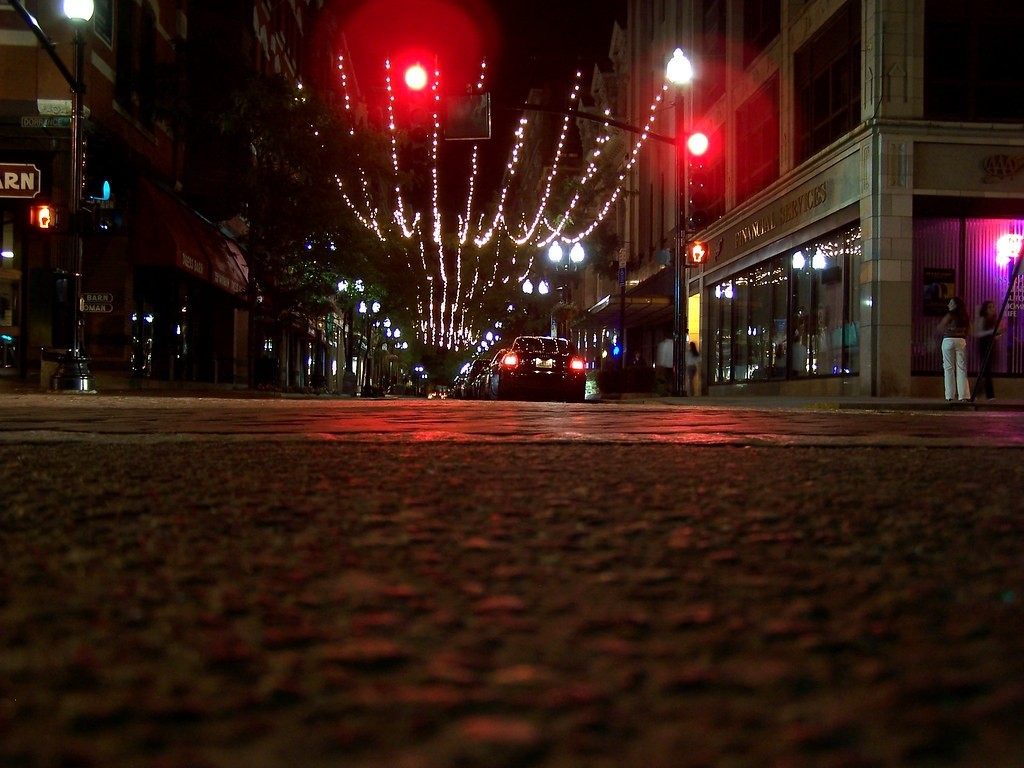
<box><xmin>936</xmin><ymin>297</ymin><xmax>972</xmax><ymax>404</ymax></box>
<box><xmin>973</xmin><ymin>300</ymin><xmax>1006</xmax><ymax>402</ymax></box>
<box><xmin>657</xmin><ymin>328</ymin><xmax>674</xmax><ymax>385</ymax></box>
<box><xmin>685</xmin><ymin>342</ymin><xmax>700</xmax><ymax>397</ymax></box>
<box><xmin>774</xmin><ymin>335</ymin><xmax>807</xmax><ymax>374</ymax></box>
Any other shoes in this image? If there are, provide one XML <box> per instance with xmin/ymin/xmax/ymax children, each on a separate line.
<box><xmin>946</xmin><ymin>399</ymin><xmax>953</xmax><ymax>401</ymax></box>
<box><xmin>961</xmin><ymin>399</ymin><xmax>968</xmax><ymax>402</ymax></box>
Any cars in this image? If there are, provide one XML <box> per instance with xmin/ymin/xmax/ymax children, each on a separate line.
<box><xmin>449</xmin><ymin>334</ymin><xmax>585</xmax><ymax>402</ymax></box>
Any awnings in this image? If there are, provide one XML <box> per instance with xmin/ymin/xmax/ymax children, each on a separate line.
<box><xmin>130</xmin><ymin>168</ymin><xmax>265</xmax><ymax>305</ymax></box>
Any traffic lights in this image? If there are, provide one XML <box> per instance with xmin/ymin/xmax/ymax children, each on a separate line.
<box><xmin>685</xmin><ymin>239</ymin><xmax>712</xmax><ymax>266</ymax></box>
<box><xmin>30</xmin><ymin>200</ymin><xmax>57</xmax><ymax>231</ymax></box>
<box><xmin>680</xmin><ymin>126</ymin><xmax>717</xmax><ymax>162</ymax></box>
<box><xmin>400</xmin><ymin>49</ymin><xmax>438</xmax><ymax>130</ymax></box>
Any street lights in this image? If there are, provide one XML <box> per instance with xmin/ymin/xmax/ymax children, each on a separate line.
<box><xmin>48</xmin><ymin>0</ymin><xmax>95</xmax><ymax>396</ymax></box>
<box><xmin>665</xmin><ymin>48</ymin><xmax>693</xmax><ymax>399</ymax></box>
<box><xmin>548</xmin><ymin>241</ymin><xmax>585</xmax><ymax>339</ymax></box>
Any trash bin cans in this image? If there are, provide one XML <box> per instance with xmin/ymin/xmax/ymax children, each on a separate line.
<box><xmin>41</xmin><ymin>352</ymin><xmax>63</xmax><ymax>388</ymax></box>
